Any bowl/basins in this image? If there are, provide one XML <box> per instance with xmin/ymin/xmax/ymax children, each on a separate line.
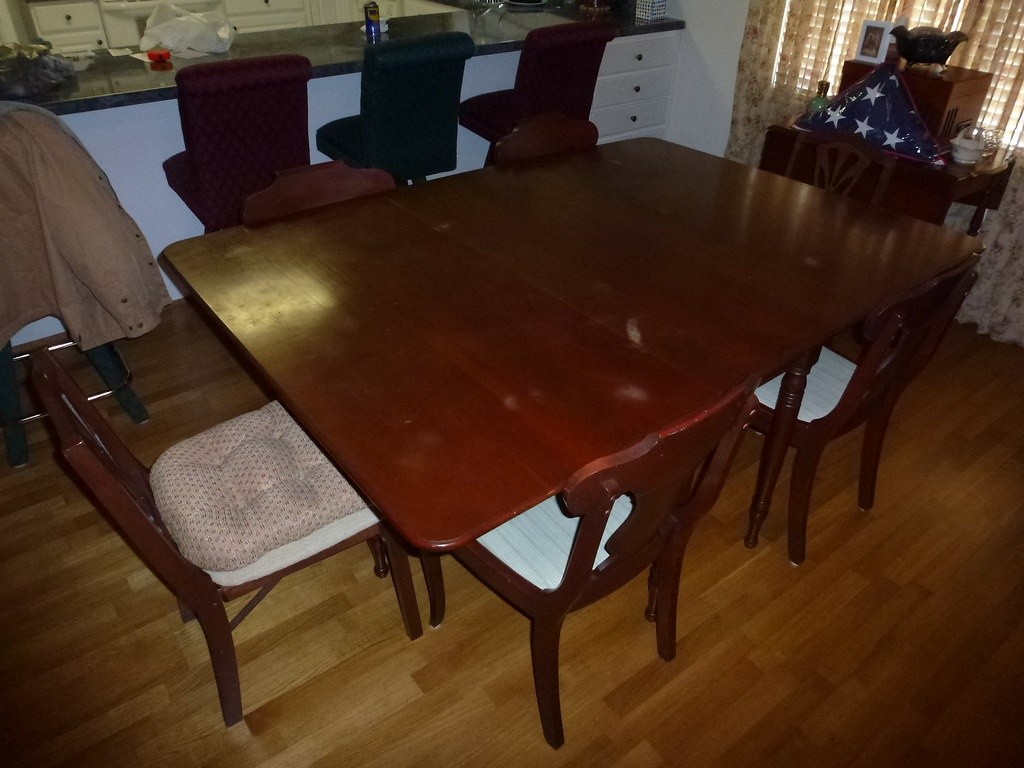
<box><xmin>950</xmin><ymin>139</ymin><xmax>984</xmax><ymax>165</ymax></box>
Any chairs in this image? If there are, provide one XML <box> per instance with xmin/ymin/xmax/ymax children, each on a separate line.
<box><xmin>242</xmin><ymin>160</ymin><xmax>397</xmax><ymax>228</ymax></box>
<box><xmin>164</xmin><ymin>54</ymin><xmax>313</xmax><ymax>234</ymax></box>
<box><xmin>457</xmin><ymin>20</ymin><xmax>618</xmax><ymax>143</ymax></box>
<box><xmin>483</xmin><ymin>111</ymin><xmax>600</xmax><ymax>167</ymax></box>
<box><xmin>694</xmin><ymin>251</ymin><xmax>982</xmax><ymax>565</ymax></box>
<box><xmin>418</xmin><ymin>374</ymin><xmax>763</xmax><ymax>750</ymax></box>
<box><xmin>25</xmin><ymin>344</ymin><xmax>446</xmax><ymax>732</ymax></box>
<box><xmin>785</xmin><ymin>127</ymin><xmax>898</xmax><ymax>205</ymax></box>
<box><xmin>317</xmin><ymin>32</ymin><xmax>476</xmax><ymax>188</ymax></box>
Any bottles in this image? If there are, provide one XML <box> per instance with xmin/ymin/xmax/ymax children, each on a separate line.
<box><xmin>806</xmin><ymin>81</ymin><xmax>829</xmax><ymax>112</ymax></box>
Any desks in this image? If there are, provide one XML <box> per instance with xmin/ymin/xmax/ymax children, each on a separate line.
<box><xmin>158</xmin><ymin>138</ymin><xmax>988</xmax><ymax>631</ymax></box>
<box><xmin>761</xmin><ymin>112</ymin><xmax>1016</xmax><ymax>236</ymax></box>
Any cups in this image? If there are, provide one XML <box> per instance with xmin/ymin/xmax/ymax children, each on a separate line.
<box><xmin>981</xmin><ymin>127</ymin><xmax>1004</xmax><ymax>159</ymax></box>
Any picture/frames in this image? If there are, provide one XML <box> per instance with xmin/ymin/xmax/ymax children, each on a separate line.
<box><xmin>853</xmin><ymin>19</ymin><xmax>894</xmax><ymax>64</ymax></box>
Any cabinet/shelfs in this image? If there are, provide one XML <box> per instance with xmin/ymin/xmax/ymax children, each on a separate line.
<box><xmin>587</xmin><ymin>32</ymin><xmax>678</xmax><ymax>145</ymax></box>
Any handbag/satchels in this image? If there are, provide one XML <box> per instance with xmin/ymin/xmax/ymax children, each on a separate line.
<box><xmin>140</xmin><ymin>2</ymin><xmax>236</xmax><ymax>53</ymax></box>
